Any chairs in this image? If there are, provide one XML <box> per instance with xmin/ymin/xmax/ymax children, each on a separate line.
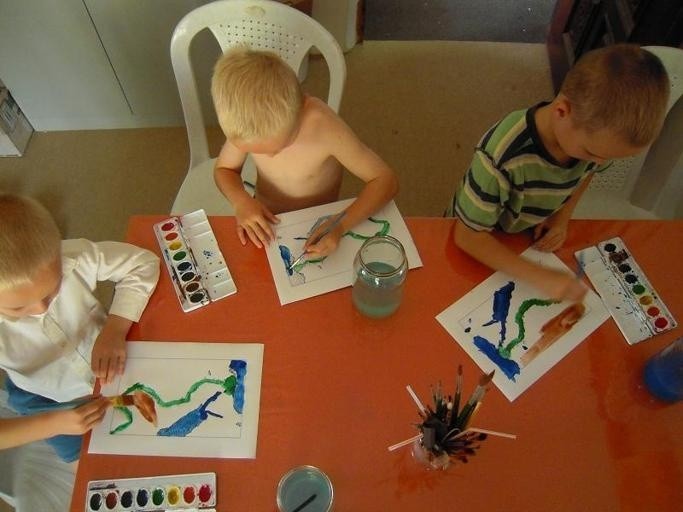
<box><xmin>565</xmin><ymin>42</ymin><xmax>683</xmax><ymax>218</ymax></box>
<box><xmin>169</xmin><ymin>0</ymin><xmax>346</xmax><ymax>215</ymax></box>
<box><xmin>0</xmin><ymin>389</ymin><xmax>80</xmax><ymax>512</ymax></box>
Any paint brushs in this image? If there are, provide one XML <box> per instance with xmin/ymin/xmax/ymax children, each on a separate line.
<box><xmin>27</xmin><ymin>395</ymin><xmax>136</xmax><ymax>412</ymax></box>
<box><xmin>288</xmin><ymin>211</ymin><xmax>346</xmax><ymax>270</ymax></box>
<box><xmin>388</xmin><ymin>363</ymin><xmax>520</xmax><ymax>470</ymax></box>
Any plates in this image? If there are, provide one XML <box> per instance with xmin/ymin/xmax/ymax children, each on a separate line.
<box><xmin>275</xmin><ymin>465</ymin><xmax>334</xmax><ymax>512</ymax></box>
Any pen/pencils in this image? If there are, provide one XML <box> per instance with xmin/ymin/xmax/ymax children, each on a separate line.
<box><xmin>244</xmin><ymin>181</ymin><xmax>256</xmax><ymax>190</ymax></box>
<box><xmin>292</xmin><ymin>494</ymin><xmax>317</xmax><ymax>512</ymax></box>
<box><xmin>575</xmin><ymin>252</ymin><xmax>585</xmax><ymax>282</ymax></box>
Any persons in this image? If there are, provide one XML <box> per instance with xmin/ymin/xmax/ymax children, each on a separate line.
<box><xmin>440</xmin><ymin>45</ymin><xmax>671</xmax><ymax>304</ymax></box>
<box><xmin>0</xmin><ymin>190</ymin><xmax>161</xmax><ymax>477</ymax></box>
<box><xmin>209</xmin><ymin>44</ymin><xmax>399</xmax><ymax>260</ymax></box>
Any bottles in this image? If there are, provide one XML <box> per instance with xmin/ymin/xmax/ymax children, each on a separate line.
<box><xmin>353</xmin><ymin>234</ymin><xmax>408</xmax><ymax>320</ymax></box>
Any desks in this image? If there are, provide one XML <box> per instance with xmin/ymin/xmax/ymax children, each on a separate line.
<box><xmin>67</xmin><ymin>215</ymin><xmax>682</xmax><ymax>512</ymax></box>
